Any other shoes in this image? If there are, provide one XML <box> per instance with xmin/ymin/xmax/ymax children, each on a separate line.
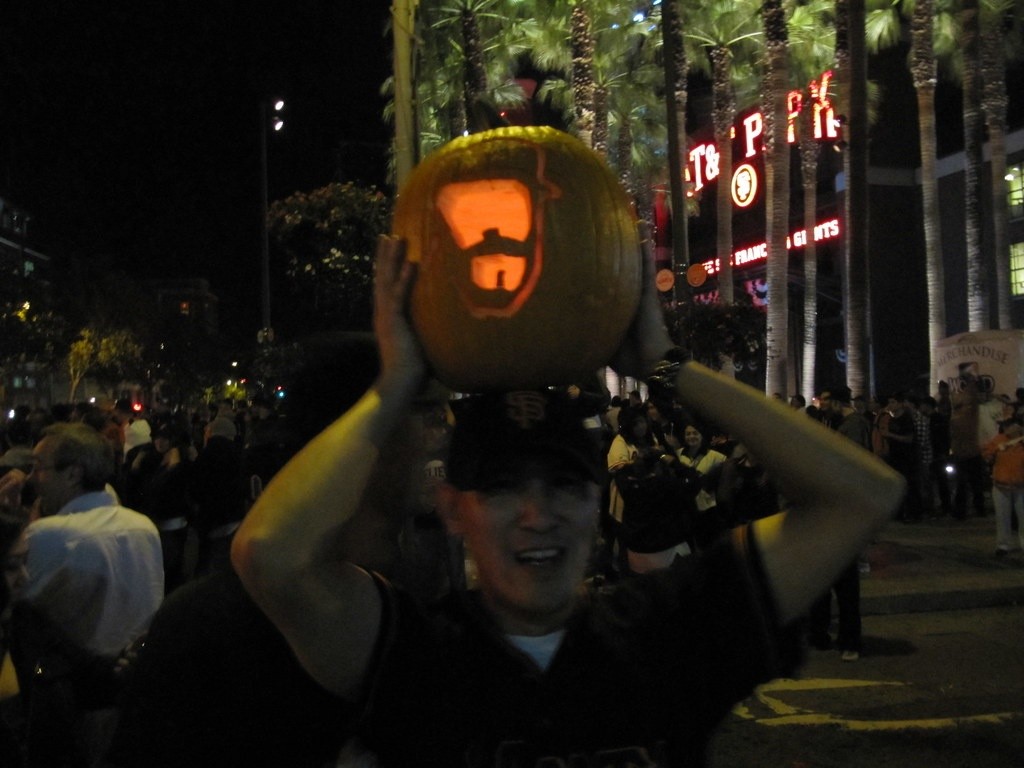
<box><xmin>996</xmin><ymin>550</ymin><xmax>1008</xmax><ymax>556</ymax></box>
<box><xmin>903</xmin><ymin>515</ymin><xmax>917</xmax><ymax>522</ymax></box>
<box><xmin>838</xmin><ymin>635</ymin><xmax>859</xmax><ymax>651</ymax></box>
<box><xmin>806</xmin><ymin>635</ymin><xmax>831</xmax><ymax>650</ymax></box>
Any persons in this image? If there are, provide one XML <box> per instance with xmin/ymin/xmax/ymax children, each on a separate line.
<box><xmin>233</xmin><ymin>232</ymin><xmax>905</xmax><ymax>768</ymax></box>
<box><xmin>0</xmin><ymin>380</ymin><xmax>1024</xmax><ymax>768</ymax></box>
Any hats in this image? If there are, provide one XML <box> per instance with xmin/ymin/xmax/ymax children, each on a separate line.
<box><xmin>996</xmin><ymin>417</ymin><xmax>1024</xmax><ymax>428</ymax></box>
<box><xmin>151</xmin><ymin>422</ymin><xmax>180</xmax><ymax>440</ymax></box>
<box><xmin>209</xmin><ymin>417</ymin><xmax>237</xmax><ymax>436</ymax></box>
<box><xmin>446</xmin><ymin>389</ymin><xmax>610</xmax><ymax>491</ymax></box>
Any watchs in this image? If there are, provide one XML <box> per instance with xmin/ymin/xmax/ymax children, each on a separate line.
<box><xmin>648</xmin><ymin>345</ymin><xmax>689</xmax><ymax>400</ymax></box>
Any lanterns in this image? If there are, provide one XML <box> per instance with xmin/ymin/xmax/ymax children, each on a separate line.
<box><xmin>391</xmin><ymin>126</ymin><xmax>639</xmax><ymax>384</ymax></box>
